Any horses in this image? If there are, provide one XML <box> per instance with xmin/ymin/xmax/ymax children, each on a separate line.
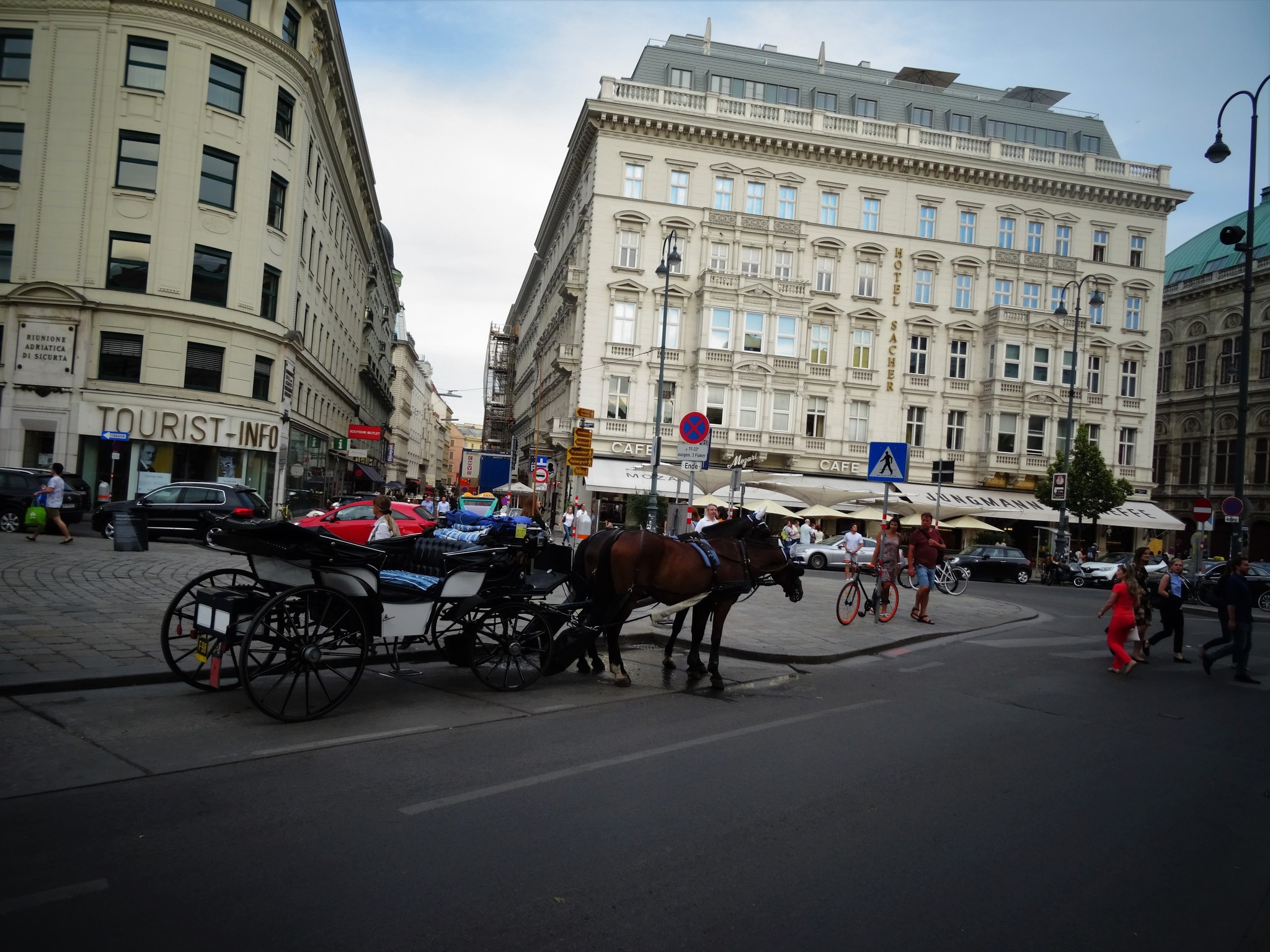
<box><xmin>570</xmin><ymin>503</ymin><xmax>804</xmax><ymax>690</ymax></box>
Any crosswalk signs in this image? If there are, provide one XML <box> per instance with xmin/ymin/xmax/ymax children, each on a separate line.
<box><xmin>867</xmin><ymin>442</ymin><xmax>907</xmax><ymax>483</ymax></box>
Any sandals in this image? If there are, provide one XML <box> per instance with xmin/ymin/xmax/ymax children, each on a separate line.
<box><xmin>917</xmin><ymin>615</ymin><xmax>935</xmax><ymax>625</ymax></box>
<box><xmin>911</xmin><ymin>607</ymin><xmax>919</xmax><ymax>620</ymax></box>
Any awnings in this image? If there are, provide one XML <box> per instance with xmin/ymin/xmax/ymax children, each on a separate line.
<box><xmin>893</xmin><ymin>482</ymin><xmax>1186</xmax><ymax>530</ymax></box>
<box><xmin>584</xmin><ymin>457</ymin><xmax>910</xmax><ymax>514</ymax></box>
<box><xmin>356</xmin><ymin>463</ymin><xmax>386</xmax><ymax>482</ymax></box>
<box><xmin>411</xmin><ymin>480</ymin><xmax>435</xmax><ymax>489</ymax></box>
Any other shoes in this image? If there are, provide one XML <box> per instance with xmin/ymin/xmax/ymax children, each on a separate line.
<box><xmin>850</xmin><ymin>578</ymin><xmax>854</xmax><ymax>581</ymax></box>
<box><xmin>59</xmin><ymin>538</ymin><xmax>73</xmax><ymax>545</ymax></box>
<box><xmin>25</xmin><ymin>536</ymin><xmax>36</xmax><ymax>542</ymax></box>
<box><xmin>1107</xmin><ymin>641</ymin><xmax>1261</xmax><ymax>682</ymax></box>
<box><xmin>846</xmin><ymin>579</ymin><xmax>849</xmax><ymax>580</ymax></box>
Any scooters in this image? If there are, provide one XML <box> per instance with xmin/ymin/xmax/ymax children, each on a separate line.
<box><xmin>1040</xmin><ymin>549</ymin><xmax>1086</xmax><ymax>588</ymax></box>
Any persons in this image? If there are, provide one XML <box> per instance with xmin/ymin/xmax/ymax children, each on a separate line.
<box><xmin>842</xmin><ymin>524</ymin><xmax>863</xmax><ymax>580</ymax></box>
<box><xmin>680</xmin><ymin>501</ymin><xmax>727</xmax><ymax>533</ymax></box>
<box><xmin>779</xmin><ymin>519</ymin><xmax>824</xmax><ymax>549</ymax></box>
<box><xmin>386</xmin><ymin>491</ymin><xmax>457</xmax><ymax>528</ymax></box>
<box><xmin>137</xmin><ymin>441</ymin><xmax>157</xmax><ymax>473</ymax></box>
<box><xmin>870</xmin><ymin>512</ymin><xmax>946</xmax><ymax>624</ymax></box>
<box><xmin>605</xmin><ymin>519</ymin><xmax>613</xmax><ymax>528</ymax></box>
<box><xmin>1097</xmin><ymin>547</ymin><xmax>1261</xmax><ymax>684</ymax></box>
<box><xmin>1052</xmin><ymin>543</ymin><xmax>1098</xmax><ymax>565</ymax></box>
<box><xmin>25</xmin><ymin>463</ymin><xmax>73</xmax><ymax>545</ymax></box>
<box><xmin>520</xmin><ymin>495</ymin><xmax>546</xmax><ymax>531</ymax></box>
<box><xmin>307</xmin><ymin>489</ymin><xmax>316</xmax><ymax>497</ymax></box>
<box><xmin>558</xmin><ymin>503</ymin><xmax>588</xmax><ymax>547</ymax></box>
<box><xmin>995</xmin><ymin>536</ymin><xmax>1006</xmax><ymax>546</ymax></box>
<box><xmin>368</xmin><ymin>495</ymin><xmax>401</xmax><ymax>541</ymax></box>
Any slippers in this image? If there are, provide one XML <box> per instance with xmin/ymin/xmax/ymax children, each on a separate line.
<box><xmin>880</xmin><ymin>613</ymin><xmax>890</xmax><ymax>617</ymax></box>
<box><xmin>870</xmin><ymin>607</ymin><xmax>874</xmax><ymax>614</ymax></box>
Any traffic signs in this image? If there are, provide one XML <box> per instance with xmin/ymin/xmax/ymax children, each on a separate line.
<box><xmin>566</xmin><ymin>427</ymin><xmax>594</xmax><ymax>477</ymax></box>
<box><xmin>1051</xmin><ymin>473</ymin><xmax>1067</xmax><ymax>501</ymax></box>
<box><xmin>576</xmin><ymin>407</ymin><xmax>594</xmax><ymax>420</ymax></box>
<box><xmin>101</xmin><ymin>430</ymin><xmax>128</xmax><ymax>441</ymax></box>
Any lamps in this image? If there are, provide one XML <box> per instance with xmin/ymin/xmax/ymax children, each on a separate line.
<box><xmin>382</xmin><ymin>421</ymin><xmax>393</xmax><ymax>430</ymax></box>
<box><xmin>1101</xmin><ymin>525</ymin><xmax>1113</xmax><ymax>538</ymax></box>
<box><xmin>280</xmin><ymin>416</ymin><xmax>290</xmax><ymax>425</ymax></box>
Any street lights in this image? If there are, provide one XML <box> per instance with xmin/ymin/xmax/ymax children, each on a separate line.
<box><xmin>1204</xmin><ymin>75</ymin><xmax>1270</xmax><ymax>565</ymax></box>
<box><xmin>645</xmin><ymin>228</ymin><xmax>682</xmax><ymax>534</ymax></box>
<box><xmin>1052</xmin><ymin>274</ymin><xmax>1104</xmax><ymax>585</ymax></box>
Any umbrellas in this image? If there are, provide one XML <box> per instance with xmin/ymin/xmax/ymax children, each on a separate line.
<box><xmin>493</xmin><ymin>482</ymin><xmax>538</xmax><ymax>508</ymax></box>
<box><xmin>382</xmin><ymin>481</ymin><xmax>408</xmax><ymax>491</ymax></box>
<box><xmin>424</xmin><ymin>491</ymin><xmax>435</xmax><ymax>494</ymax></box>
<box><xmin>691</xmin><ymin>492</ymin><xmax>1004</xmax><ymax>531</ymax></box>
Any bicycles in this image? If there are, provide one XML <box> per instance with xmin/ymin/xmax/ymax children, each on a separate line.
<box><xmin>274</xmin><ymin>502</ymin><xmax>295</xmax><ymax>523</ymax></box>
<box><xmin>1180</xmin><ymin>568</ymin><xmax>1218</xmax><ymax>607</ymax></box>
<box><xmin>836</xmin><ymin>559</ymin><xmax>899</xmax><ymax>625</ymax></box>
<box><xmin>543</xmin><ymin>517</ymin><xmax>555</xmax><ymax>543</ymax></box>
<box><xmin>897</xmin><ymin>556</ymin><xmax>969</xmax><ymax>596</ymax></box>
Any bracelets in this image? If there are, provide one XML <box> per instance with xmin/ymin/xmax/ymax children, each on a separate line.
<box><xmin>606</xmin><ymin>523</ymin><xmax>607</xmax><ymax>524</ymax></box>
<box><xmin>559</xmin><ymin>524</ymin><xmax>561</xmax><ymax>525</ymax></box>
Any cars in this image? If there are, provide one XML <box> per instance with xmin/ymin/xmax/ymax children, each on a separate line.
<box><xmin>944</xmin><ymin>544</ymin><xmax>1033</xmax><ymax>585</ymax></box>
<box><xmin>290</xmin><ymin>491</ymin><xmax>522</xmax><ymax>546</ymax></box>
<box><xmin>288</xmin><ymin>489</ymin><xmax>321</xmax><ymax>510</ymax></box>
<box><xmin>786</xmin><ymin>535</ymin><xmax>918</xmax><ymax>575</ymax></box>
<box><xmin>1250</xmin><ymin>562</ymin><xmax>1270</xmax><ymax>571</ymax></box>
<box><xmin>1146</xmin><ymin>558</ymin><xmax>1270</xmax><ymax>612</ymax></box>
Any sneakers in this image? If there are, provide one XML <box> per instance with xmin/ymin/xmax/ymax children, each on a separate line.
<box><xmin>561</xmin><ymin>542</ymin><xmax>565</xmax><ymax>546</ymax></box>
<box><xmin>568</xmin><ymin>544</ymin><xmax>571</xmax><ymax>547</ymax></box>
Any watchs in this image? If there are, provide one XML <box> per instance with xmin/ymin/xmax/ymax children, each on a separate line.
<box><xmin>855</xmin><ymin>549</ymin><xmax>857</xmax><ymax>552</ymax></box>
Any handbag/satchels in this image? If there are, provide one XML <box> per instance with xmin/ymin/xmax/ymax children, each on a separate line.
<box><xmin>24</xmin><ymin>496</ymin><xmax>46</xmax><ymax>527</ymax></box>
<box><xmin>562</xmin><ymin>513</ymin><xmax>567</xmax><ymax>523</ymax></box>
<box><xmin>810</xmin><ymin>533</ymin><xmax>816</xmax><ymax>543</ymax></box>
<box><xmin>1087</xmin><ymin>552</ymin><xmax>1093</xmax><ymax>559</ymax></box>
<box><xmin>1124</xmin><ymin>625</ymin><xmax>1140</xmax><ymax>641</ymax></box>
<box><xmin>781</xmin><ymin>526</ymin><xmax>792</xmax><ymax>540</ymax></box>
<box><xmin>34</xmin><ymin>488</ymin><xmax>47</xmax><ymax>508</ymax></box>
<box><xmin>935</xmin><ymin>549</ymin><xmax>945</xmax><ymax>565</ymax></box>
<box><xmin>1074</xmin><ymin>557</ymin><xmax>1079</xmax><ymax>563</ymax></box>
<box><xmin>1149</xmin><ymin>573</ymin><xmax>1183</xmax><ymax>613</ymax></box>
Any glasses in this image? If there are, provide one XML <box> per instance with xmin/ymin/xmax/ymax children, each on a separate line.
<box><xmin>708</xmin><ymin>509</ymin><xmax>717</xmax><ymax>511</ymax></box>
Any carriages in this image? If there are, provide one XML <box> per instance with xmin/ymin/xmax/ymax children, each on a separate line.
<box><xmin>161</xmin><ymin>510</ymin><xmax>804</xmax><ymax>725</ymax></box>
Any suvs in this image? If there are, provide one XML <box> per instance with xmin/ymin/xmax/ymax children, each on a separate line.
<box><xmin>0</xmin><ymin>467</ymin><xmax>93</xmax><ymax>533</ymax></box>
<box><xmin>91</xmin><ymin>482</ymin><xmax>271</xmax><ymax>547</ymax></box>
<box><xmin>1080</xmin><ymin>552</ymin><xmax>1168</xmax><ymax>591</ymax></box>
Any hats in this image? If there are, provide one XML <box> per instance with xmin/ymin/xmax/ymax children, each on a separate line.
<box><xmin>1093</xmin><ymin>543</ymin><xmax>1098</xmax><ymax>547</ymax></box>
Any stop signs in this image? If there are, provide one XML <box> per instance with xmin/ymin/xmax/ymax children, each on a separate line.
<box><xmin>1193</xmin><ymin>498</ymin><xmax>1212</xmax><ymax>522</ymax></box>
